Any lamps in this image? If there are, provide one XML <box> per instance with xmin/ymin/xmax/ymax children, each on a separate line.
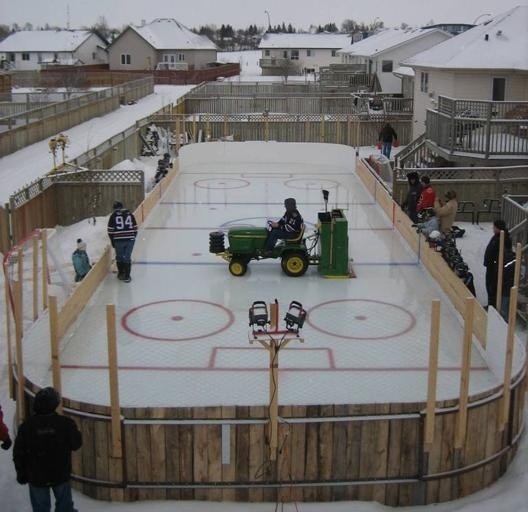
<box><xmin>249</xmin><ymin>297</ymin><xmax>305</xmax><ymax>341</ymax></box>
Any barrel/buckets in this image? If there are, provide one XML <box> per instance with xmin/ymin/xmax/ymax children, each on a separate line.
<box><xmin>377</xmin><ymin>143</ymin><xmax>381</xmax><ymax>149</ymax></box>
<box><xmin>393</xmin><ymin>141</ymin><xmax>400</xmax><ymax>147</ymax></box>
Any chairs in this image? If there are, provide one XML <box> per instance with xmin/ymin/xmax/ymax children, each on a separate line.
<box><xmin>282</xmin><ymin>221</ymin><xmax>306</xmax><ymax>242</ymax></box>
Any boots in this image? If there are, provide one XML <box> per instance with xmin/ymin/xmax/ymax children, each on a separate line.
<box><xmin>115</xmin><ymin>260</ymin><xmax>131</xmax><ymax>281</ymax></box>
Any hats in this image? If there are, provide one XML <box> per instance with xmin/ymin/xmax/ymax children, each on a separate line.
<box><xmin>493</xmin><ymin>219</ymin><xmax>510</xmax><ymax>232</ymax></box>
<box><xmin>77</xmin><ymin>242</ymin><xmax>86</xmax><ymax>249</ymax></box>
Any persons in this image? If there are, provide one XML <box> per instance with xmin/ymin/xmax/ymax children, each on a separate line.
<box><xmin>154</xmin><ymin>153</ymin><xmax>174</xmax><ymax>183</ymax></box>
<box><xmin>264</xmin><ymin>197</ymin><xmax>304</xmax><ymax>250</ymax></box>
<box><xmin>376</xmin><ymin>122</ymin><xmax>397</xmax><ymax>159</ymax></box>
<box><xmin>401</xmin><ymin>172</ymin><xmax>477</xmax><ymax>297</ymax></box>
<box><xmin>13</xmin><ymin>387</ymin><xmax>83</xmax><ymax>512</ymax></box>
<box><xmin>483</xmin><ymin>220</ymin><xmax>516</xmax><ymax>310</ymax></box>
<box><xmin>107</xmin><ymin>199</ymin><xmax>138</xmax><ymax>282</ymax></box>
<box><xmin>0</xmin><ymin>405</ymin><xmax>12</xmax><ymax>450</ymax></box>
<box><xmin>71</xmin><ymin>238</ymin><xmax>92</xmax><ymax>282</ymax></box>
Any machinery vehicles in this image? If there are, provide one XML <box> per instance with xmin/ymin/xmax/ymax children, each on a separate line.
<box><xmin>209</xmin><ymin>189</ymin><xmax>357</xmax><ymax>280</ymax></box>
